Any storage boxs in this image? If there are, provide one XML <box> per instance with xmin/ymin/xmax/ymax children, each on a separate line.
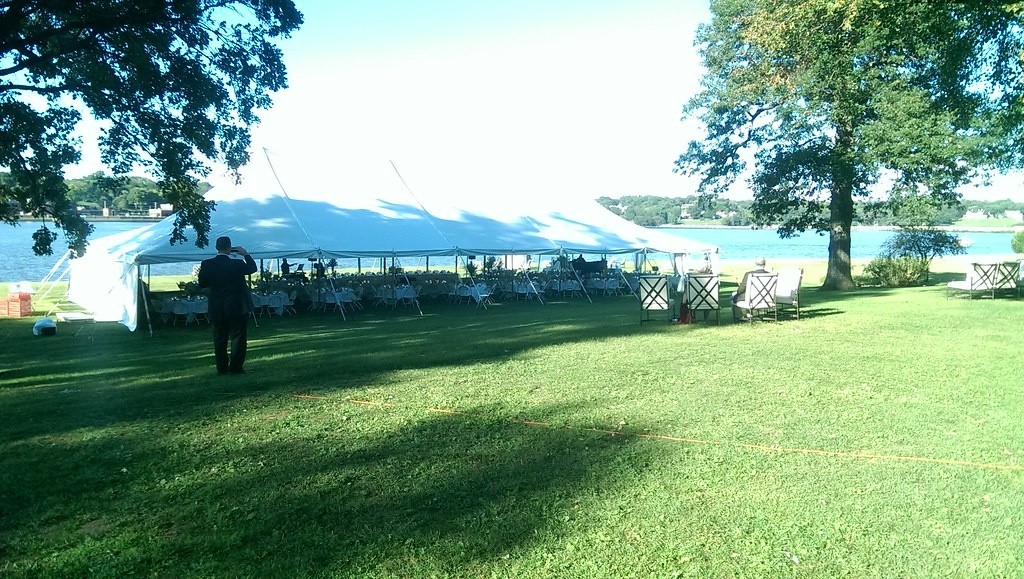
<box><xmin>0</xmin><ymin>292</ymin><xmax>31</xmax><ymax>316</ymax></box>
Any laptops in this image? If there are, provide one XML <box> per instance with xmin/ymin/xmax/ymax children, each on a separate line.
<box><xmin>297</xmin><ymin>264</ymin><xmax>304</xmax><ymax>270</ymax></box>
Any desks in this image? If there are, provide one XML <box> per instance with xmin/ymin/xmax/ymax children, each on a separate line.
<box><xmin>170</xmin><ymin>270</ymin><xmax>620</xmax><ymax>304</ymax></box>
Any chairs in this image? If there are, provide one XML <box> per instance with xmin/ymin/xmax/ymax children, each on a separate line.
<box><xmin>147</xmin><ymin>269</ymin><xmax>628</xmax><ymax>326</ymax></box>
<box><xmin>639</xmin><ymin>275</ymin><xmax>675</xmax><ymax>327</ymax></box>
<box><xmin>684</xmin><ymin>273</ymin><xmax>721</xmax><ymax>326</ymax></box>
<box><xmin>775</xmin><ymin>267</ymin><xmax>803</xmax><ymax>320</ymax></box>
<box><xmin>947</xmin><ymin>258</ymin><xmax>1024</xmax><ymax>300</ymax></box>
<box><xmin>729</xmin><ymin>273</ymin><xmax>779</xmax><ymax>327</ymax></box>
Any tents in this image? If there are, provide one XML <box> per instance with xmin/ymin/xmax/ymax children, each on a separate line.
<box><xmin>67</xmin><ymin>166</ymin><xmax>721</xmax><ymax>337</ymax></box>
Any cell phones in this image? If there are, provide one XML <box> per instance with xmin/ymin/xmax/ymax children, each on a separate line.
<box><xmin>231</xmin><ymin>247</ymin><xmax>239</xmax><ymax>252</ymax></box>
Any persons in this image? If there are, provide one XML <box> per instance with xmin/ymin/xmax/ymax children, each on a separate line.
<box><xmin>281</xmin><ymin>258</ymin><xmax>296</xmax><ymax>276</ymax></box>
<box><xmin>733</xmin><ymin>256</ymin><xmax>771</xmax><ymax>318</ymax></box>
<box><xmin>577</xmin><ymin>254</ymin><xmax>587</xmax><ymax>262</ymax></box>
<box><xmin>197</xmin><ymin>236</ymin><xmax>257</xmax><ymax>375</ymax></box>
